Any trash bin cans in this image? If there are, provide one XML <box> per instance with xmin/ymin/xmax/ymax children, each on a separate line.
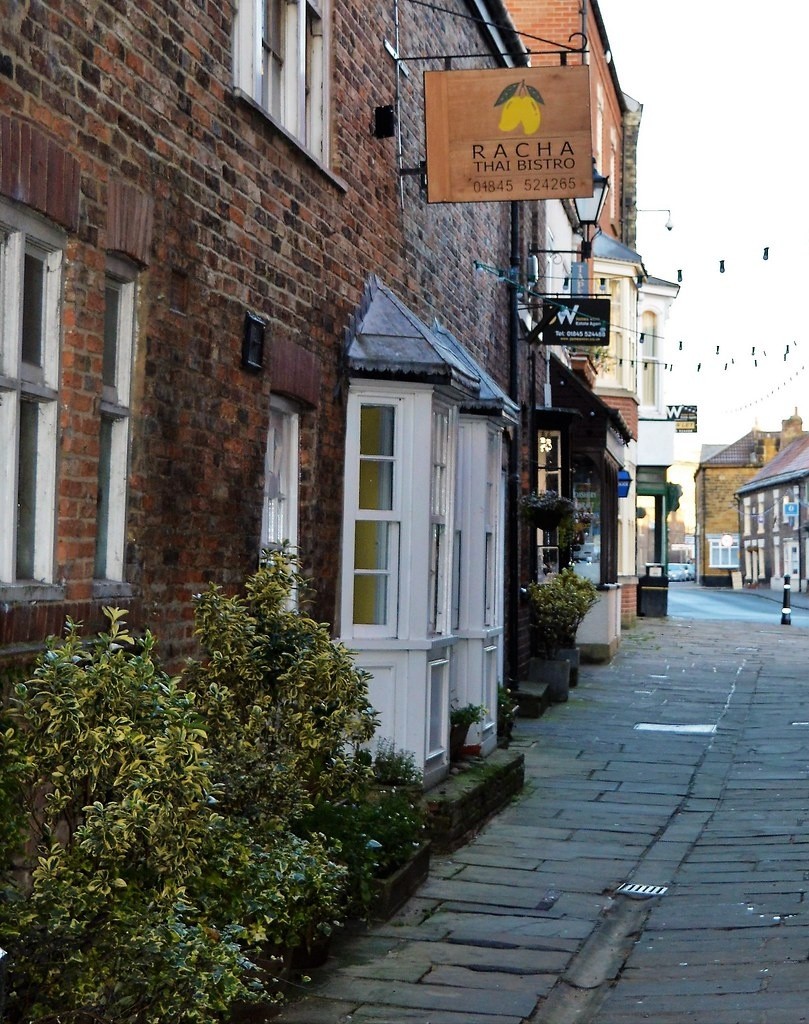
<box><xmin>639</xmin><ymin>563</ymin><xmax>669</xmax><ymax>617</ymax></box>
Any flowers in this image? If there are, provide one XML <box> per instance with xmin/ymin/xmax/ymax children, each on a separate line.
<box><xmin>450</xmin><ymin>703</ymin><xmax>490</xmax><ymax>736</ymax></box>
<box><xmin>497</xmin><ymin>682</ymin><xmax>520</xmax><ymax>723</ymax></box>
<box><xmin>518</xmin><ymin>486</ymin><xmax>574</xmax><ymax>525</ymax></box>
<box><xmin>350</xmin><ymin>801</ymin><xmax>426</xmax><ymax>881</ymax></box>
<box><xmin>367</xmin><ymin>738</ymin><xmax>424</xmax><ymax>787</ymax></box>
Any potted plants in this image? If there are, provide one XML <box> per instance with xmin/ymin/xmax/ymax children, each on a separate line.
<box><xmin>525</xmin><ymin>568</ymin><xmax>600</xmax><ymax>701</ymax></box>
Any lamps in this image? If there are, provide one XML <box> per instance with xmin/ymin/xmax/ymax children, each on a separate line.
<box><xmin>526</xmin><ymin>155</ymin><xmax>611</xmax><ymax>261</ymax></box>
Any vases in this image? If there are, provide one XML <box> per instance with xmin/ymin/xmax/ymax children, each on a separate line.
<box><xmin>449</xmin><ymin>735</ymin><xmax>464</xmax><ymax>762</ymax></box>
<box><xmin>534</xmin><ymin>510</ymin><xmax>562</xmax><ymax>533</ymax></box>
<box><xmin>367</xmin><ymin>840</ymin><xmax>435</xmax><ymax>923</ymax></box>
<box><xmin>498</xmin><ymin>722</ymin><xmax>514</xmax><ymax>736</ymax></box>
<box><xmin>367</xmin><ymin>784</ymin><xmax>422</xmax><ymax>808</ymax></box>
